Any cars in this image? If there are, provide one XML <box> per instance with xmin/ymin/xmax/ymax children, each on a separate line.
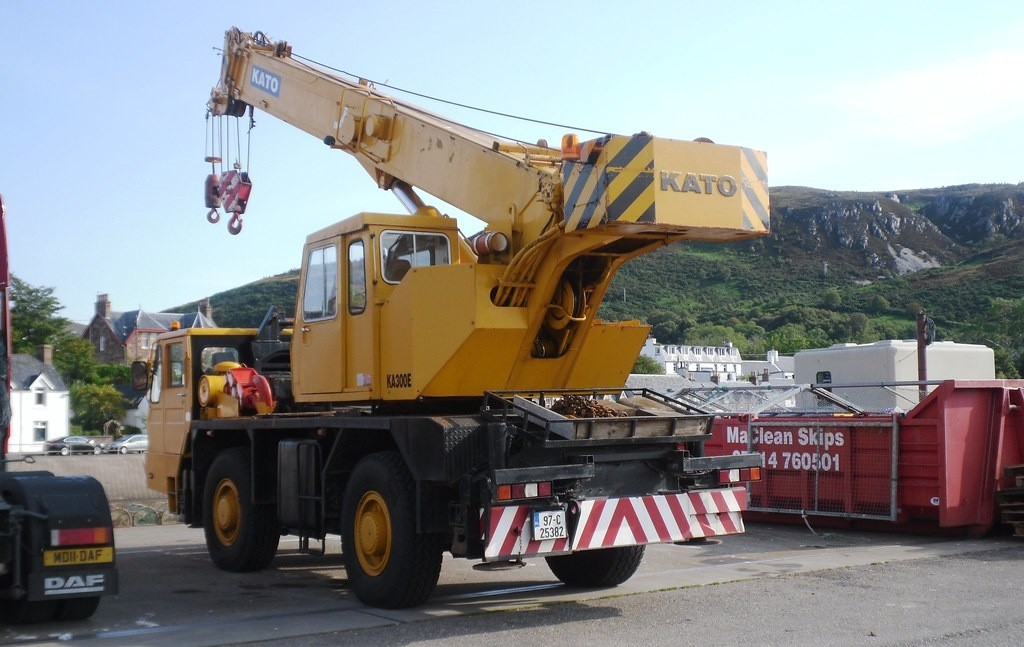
<box><xmin>106</xmin><ymin>434</ymin><xmax>148</xmax><ymax>453</ymax></box>
<box><xmin>44</xmin><ymin>436</ymin><xmax>104</xmax><ymax>455</ymax></box>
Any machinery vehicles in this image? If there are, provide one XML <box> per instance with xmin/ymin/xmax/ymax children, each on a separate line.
<box><xmin>145</xmin><ymin>27</ymin><xmax>771</xmax><ymax>610</ymax></box>
